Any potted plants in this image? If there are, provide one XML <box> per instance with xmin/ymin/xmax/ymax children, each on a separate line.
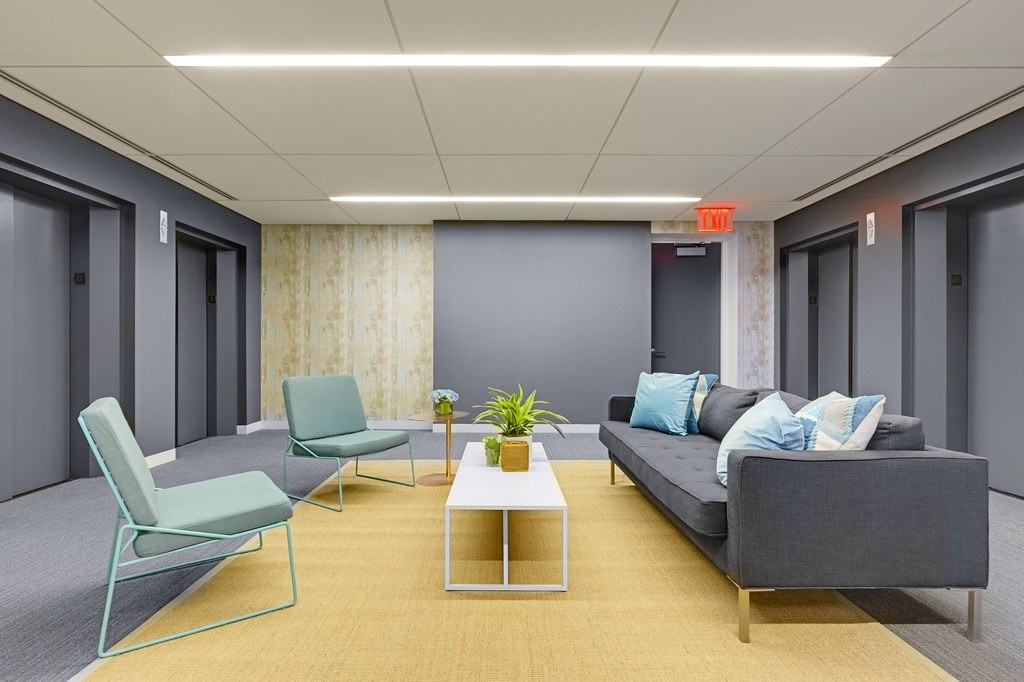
<box><xmin>472</xmin><ymin>384</ymin><xmax>570</xmax><ymax>461</ymax></box>
<box><xmin>482</xmin><ymin>438</ymin><xmax>500</xmax><ymax>467</ymax></box>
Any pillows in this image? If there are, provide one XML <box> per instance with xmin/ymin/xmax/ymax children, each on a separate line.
<box><xmin>686</xmin><ymin>373</ymin><xmax>719</xmax><ymax>432</ymax></box>
<box><xmin>630</xmin><ymin>371</ymin><xmax>700</xmax><ymax>436</ymax></box>
<box><xmin>794</xmin><ymin>390</ymin><xmax>886</xmax><ymax>450</ymax></box>
<box><xmin>716</xmin><ymin>392</ymin><xmax>805</xmax><ymax>487</ymax></box>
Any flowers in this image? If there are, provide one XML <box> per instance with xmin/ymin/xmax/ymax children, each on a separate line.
<box><xmin>428</xmin><ymin>388</ymin><xmax>459</xmax><ymax>405</ymax></box>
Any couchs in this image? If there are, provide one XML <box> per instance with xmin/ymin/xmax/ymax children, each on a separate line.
<box><xmin>599</xmin><ymin>394</ymin><xmax>989</xmax><ymax>643</ymax></box>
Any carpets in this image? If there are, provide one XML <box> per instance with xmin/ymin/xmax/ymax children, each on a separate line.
<box><xmin>75</xmin><ymin>461</ymin><xmax>957</xmax><ymax>682</ymax></box>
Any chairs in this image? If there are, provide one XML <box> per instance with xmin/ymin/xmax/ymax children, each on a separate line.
<box><xmin>78</xmin><ymin>397</ymin><xmax>297</xmax><ymax>658</ymax></box>
<box><xmin>283</xmin><ymin>375</ymin><xmax>415</xmax><ymax>512</ymax></box>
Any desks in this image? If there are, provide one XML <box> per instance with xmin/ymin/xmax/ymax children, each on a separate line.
<box><xmin>409</xmin><ymin>412</ymin><xmax>468</xmax><ymax>485</ymax></box>
<box><xmin>445</xmin><ymin>441</ymin><xmax>569</xmax><ymax>592</ymax></box>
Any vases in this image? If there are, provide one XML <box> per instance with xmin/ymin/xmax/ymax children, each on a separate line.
<box><xmin>434</xmin><ymin>401</ymin><xmax>453</xmax><ymax>415</ymax></box>
